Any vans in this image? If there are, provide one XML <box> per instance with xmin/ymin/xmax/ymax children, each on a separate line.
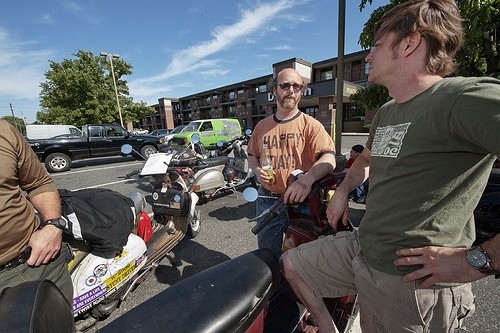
<box><xmin>173</xmin><ymin>118</ymin><xmax>242</xmax><ymax>150</ymax></box>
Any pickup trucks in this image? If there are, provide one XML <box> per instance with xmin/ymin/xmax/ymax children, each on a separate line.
<box><xmin>27</xmin><ymin>123</ymin><xmax>164</xmax><ymax>173</ymax></box>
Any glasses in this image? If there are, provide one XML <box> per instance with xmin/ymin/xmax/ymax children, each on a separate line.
<box><xmin>275</xmin><ymin>82</ymin><xmax>304</xmax><ymax>91</ymax></box>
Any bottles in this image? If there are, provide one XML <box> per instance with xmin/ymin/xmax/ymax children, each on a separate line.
<box><xmin>260</xmin><ymin>143</ymin><xmax>274</xmax><ymax>187</ymax></box>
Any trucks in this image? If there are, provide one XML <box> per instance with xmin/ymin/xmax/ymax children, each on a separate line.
<box><xmin>25</xmin><ymin>124</ymin><xmax>83</xmax><ymax>140</ymax></box>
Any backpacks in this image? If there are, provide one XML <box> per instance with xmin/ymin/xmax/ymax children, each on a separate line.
<box><xmin>33</xmin><ymin>187</ymin><xmax>136</xmax><ymax>259</ymax></box>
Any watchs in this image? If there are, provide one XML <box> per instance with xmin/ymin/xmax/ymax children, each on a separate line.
<box><xmin>467</xmin><ymin>246</ymin><xmax>495</xmax><ymax>275</ymax></box>
<box><xmin>45</xmin><ymin>218</ymin><xmax>66</xmax><ymax>230</ymax></box>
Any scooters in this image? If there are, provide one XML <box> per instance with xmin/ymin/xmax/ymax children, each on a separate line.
<box><xmin>186</xmin><ymin>127</ymin><xmax>261</xmax><ymax>206</ymax></box>
<box><xmin>65</xmin><ymin>132</ymin><xmax>201</xmax><ymax>332</ymax></box>
<box><xmin>0</xmin><ymin>171</ymin><xmax>360</xmax><ymax>333</ymax></box>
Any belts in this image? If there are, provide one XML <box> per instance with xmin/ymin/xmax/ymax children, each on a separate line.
<box><xmin>0</xmin><ymin>246</ymin><xmax>33</xmax><ymax>272</ymax></box>
<box><xmin>260</xmin><ymin>187</ymin><xmax>285</xmax><ymax>199</ymax></box>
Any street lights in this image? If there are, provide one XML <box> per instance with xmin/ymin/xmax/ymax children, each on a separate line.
<box><xmin>100</xmin><ymin>52</ymin><xmax>125</xmax><ymax>134</ymax></box>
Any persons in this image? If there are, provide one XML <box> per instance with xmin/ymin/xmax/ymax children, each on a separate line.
<box><xmin>0</xmin><ymin>118</ymin><xmax>74</xmax><ymax>307</ymax></box>
<box><xmin>344</xmin><ymin>145</ymin><xmax>365</xmax><ymax>168</ymax></box>
<box><xmin>279</xmin><ymin>0</ymin><xmax>500</xmax><ymax>333</ymax></box>
<box><xmin>247</xmin><ymin>68</ymin><xmax>337</xmax><ymax>256</ymax></box>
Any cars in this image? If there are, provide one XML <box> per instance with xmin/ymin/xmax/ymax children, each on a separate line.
<box><xmin>131</xmin><ymin>125</ymin><xmax>187</xmax><ymax>147</ymax></box>
